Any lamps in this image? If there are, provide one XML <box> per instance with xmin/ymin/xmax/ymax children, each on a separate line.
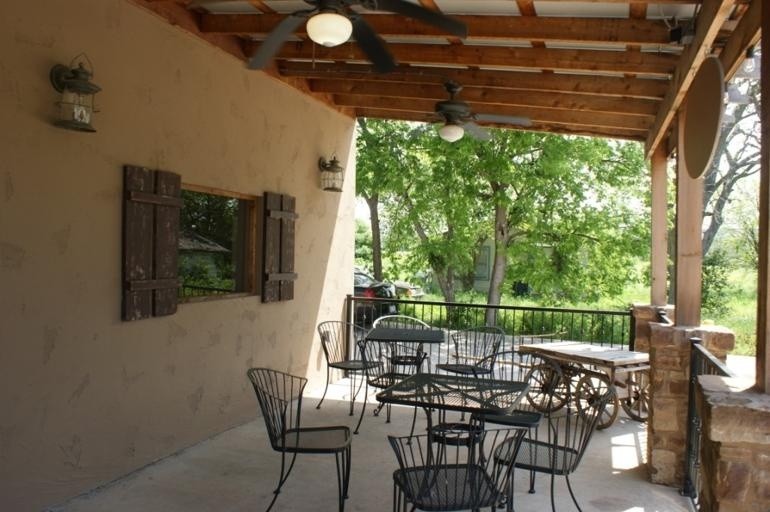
<box><xmin>51</xmin><ymin>52</ymin><xmax>100</xmax><ymax>133</ymax></box>
<box><xmin>439</xmin><ymin>118</ymin><xmax>463</xmax><ymax>141</ymax></box>
<box><xmin>742</xmin><ymin>45</ymin><xmax>755</xmax><ymax>72</ymax></box>
<box><xmin>306</xmin><ymin>9</ymin><xmax>353</xmax><ymax>48</ymax></box>
<box><xmin>320</xmin><ymin>156</ymin><xmax>345</xmax><ymax>192</ymax></box>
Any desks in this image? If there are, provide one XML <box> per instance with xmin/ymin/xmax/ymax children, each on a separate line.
<box><xmin>377</xmin><ymin>374</ymin><xmax>527</xmax><ymax>512</ymax></box>
<box><xmin>365</xmin><ymin>328</ymin><xmax>446</xmax><ymax>416</ymax></box>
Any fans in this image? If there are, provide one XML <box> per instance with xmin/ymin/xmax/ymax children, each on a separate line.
<box><xmin>247</xmin><ymin>0</ymin><xmax>469</xmax><ymax>75</ymax></box>
<box><xmin>426</xmin><ymin>77</ymin><xmax>531</xmax><ymax>143</ymax></box>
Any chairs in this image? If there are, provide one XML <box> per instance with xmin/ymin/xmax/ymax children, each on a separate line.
<box><xmin>317</xmin><ymin>320</ymin><xmax>387</xmax><ymax>417</ymax></box>
<box><xmin>372</xmin><ymin>314</ymin><xmax>430</xmax><ymax>392</ymax></box>
<box><xmin>353</xmin><ymin>339</ymin><xmax>446</xmax><ymax>444</ymax></box>
<box><xmin>469</xmin><ymin>351</ymin><xmax>566</xmax><ymax>495</ymax></box>
<box><xmin>247</xmin><ymin>367</ymin><xmax>352</xmax><ymax>511</ymax></box>
<box><xmin>386</xmin><ymin>429</ymin><xmax>527</xmax><ymax>512</ymax></box>
<box><xmin>492</xmin><ymin>365</ymin><xmax>616</xmax><ymax>512</ymax></box>
<box><xmin>432</xmin><ymin>327</ymin><xmax>504</xmax><ymax>420</ymax></box>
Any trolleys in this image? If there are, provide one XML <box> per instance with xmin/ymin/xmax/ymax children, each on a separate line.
<box><xmin>516</xmin><ymin>340</ymin><xmax>651</xmax><ymax>428</ymax></box>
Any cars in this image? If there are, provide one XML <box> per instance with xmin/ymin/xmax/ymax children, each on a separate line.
<box><xmin>390</xmin><ymin>281</ymin><xmax>423</xmax><ymax>298</ymax></box>
<box><xmin>354</xmin><ymin>271</ymin><xmax>398</xmax><ymax>321</ymax></box>
